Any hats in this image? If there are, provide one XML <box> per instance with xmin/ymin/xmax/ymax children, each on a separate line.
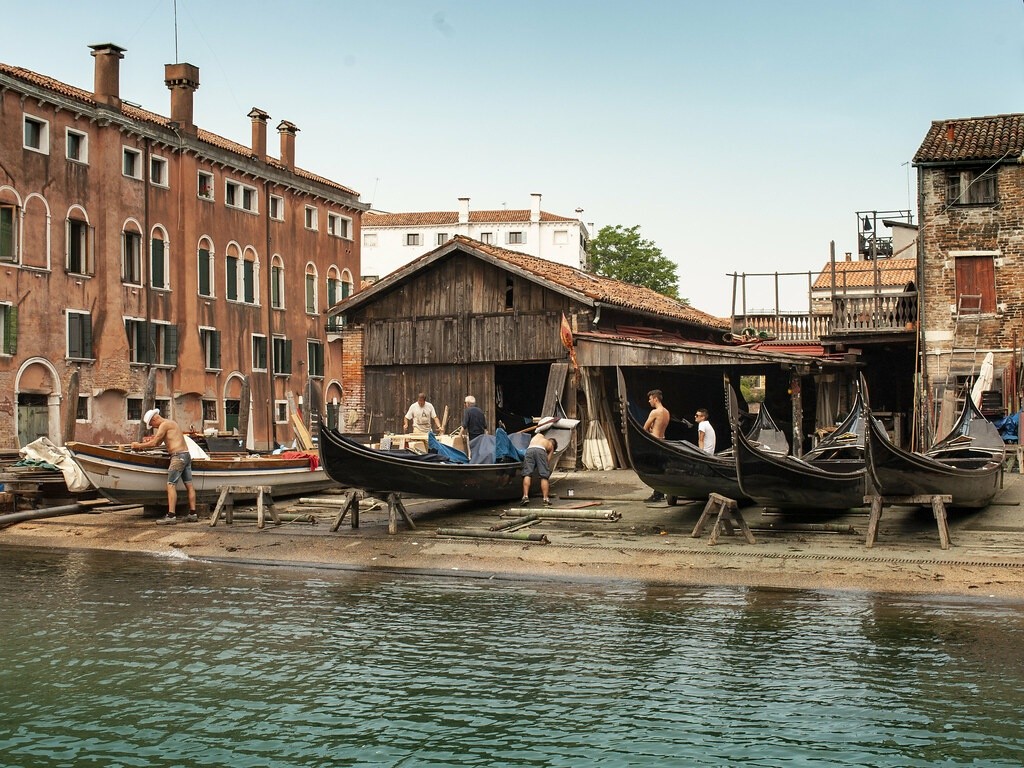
<box><xmin>143</xmin><ymin>408</ymin><xmax>160</xmax><ymax>430</ymax></box>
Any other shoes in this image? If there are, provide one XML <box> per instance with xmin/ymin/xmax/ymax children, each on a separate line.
<box><xmin>644</xmin><ymin>494</ymin><xmax>665</xmax><ymax>503</ymax></box>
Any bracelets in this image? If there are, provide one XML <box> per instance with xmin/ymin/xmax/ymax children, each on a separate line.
<box><xmin>437</xmin><ymin>426</ymin><xmax>441</xmax><ymax>428</ymax></box>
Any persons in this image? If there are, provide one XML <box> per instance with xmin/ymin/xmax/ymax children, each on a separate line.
<box><xmin>694</xmin><ymin>408</ymin><xmax>716</xmax><ymax>456</ymax></box>
<box><xmin>459</xmin><ymin>396</ymin><xmax>488</xmax><ymax>441</ymax></box>
<box><xmin>402</xmin><ymin>393</ymin><xmax>445</xmax><ymax>434</ymax></box>
<box><xmin>130</xmin><ymin>408</ymin><xmax>197</xmax><ymax>524</ymax></box>
<box><xmin>521</xmin><ymin>433</ymin><xmax>558</xmax><ymax>507</ymax></box>
<box><xmin>643</xmin><ymin>390</ymin><xmax>670</xmax><ymax>503</ymax></box>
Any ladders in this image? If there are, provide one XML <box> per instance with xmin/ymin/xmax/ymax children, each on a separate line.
<box><xmin>938</xmin><ymin>292</ymin><xmax>982</xmax><ymax>415</ymax></box>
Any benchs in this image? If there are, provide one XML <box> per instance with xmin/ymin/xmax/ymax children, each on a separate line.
<box><xmin>721</xmin><ymin>457</ymin><xmax>736</xmax><ymax>460</ymax></box>
<box><xmin>863</xmin><ymin>493</ymin><xmax>957</xmax><ymax>550</ymax></box>
<box><xmin>211</xmin><ymin>485</ymin><xmax>281</xmax><ymax>529</ymax></box>
<box><xmin>806</xmin><ymin>459</ymin><xmax>866</xmax><ymax>472</ymax></box>
<box><xmin>934</xmin><ymin>458</ymin><xmax>991</xmax><ymax>471</ymax></box>
<box><xmin>330</xmin><ymin>489</ymin><xmax>417</xmax><ymax>534</ymax></box>
<box><xmin>690</xmin><ymin>493</ymin><xmax>755</xmax><ymax>546</ymax></box>
<box><xmin>1005</xmin><ymin>444</ymin><xmax>1024</xmax><ymax>474</ymax></box>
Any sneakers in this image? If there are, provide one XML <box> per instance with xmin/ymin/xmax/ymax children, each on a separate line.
<box><xmin>182</xmin><ymin>514</ymin><xmax>197</xmax><ymax>522</ymax></box>
<box><xmin>542</xmin><ymin>498</ymin><xmax>552</xmax><ymax>506</ymax></box>
<box><xmin>156</xmin><ymin>514</ymin><xmax>177</xmax><ymax>525</ymax></box>
<box><xmin>520</xmin><ymin>497</ymin><xmax>529</xmax><ymax>506</ymax></box>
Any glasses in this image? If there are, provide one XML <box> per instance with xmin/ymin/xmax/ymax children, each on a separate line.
<box><xmin>695</xmin><ymin>413</ymin><xmax>704</xmax><ymax>418</ymax></box>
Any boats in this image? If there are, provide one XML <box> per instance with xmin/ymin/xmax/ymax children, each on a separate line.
<box><xmin>313</xmin><ymin>390</ymin><xmax>573</xmax><ymax>499</ymax></box>
<box><xmin>614</xmin><ymin>362</ymin><xmax>870</xmax><ymax>511</ymax></box>
<box><xmin>856</xmin><ymin>382</ymin><xmax>1007</xmax><ymax>508</ymax></box>
<box><xmin>65</xmin><ymin>440</ymin><xmax>330</xmax><ymax>504</ymax></box>
